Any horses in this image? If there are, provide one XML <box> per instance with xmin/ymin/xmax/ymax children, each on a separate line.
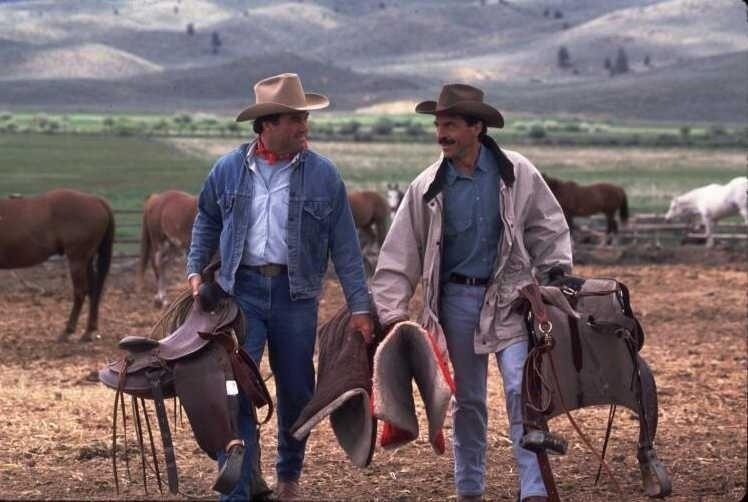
<box><xmin>663</xmin><ymin>176</ymin><xmax>748</xmax><ymax>249</ymax></box>
<box><xmin>539</xmin><ymin>172</ymin><xmax>628</xmax><ymax>246</ymax></box>
<box><xmin>384</xmin><ymin>182</ymin><xmax>405</xmax><ymax>211</ymax></box>
<box><xmin>0</xmin><ymin>186</ymin><xmax>115</xmax><ymax>345</ymax></box>
<box><xmin>346</xmin><ymin>190</ymin><xmax>391</xmax><ymax>259</ymax></box>
<box><xmin>138</xmin><ymin>188</ymin><xmax>200</xmax><ymax>309</ymax></box>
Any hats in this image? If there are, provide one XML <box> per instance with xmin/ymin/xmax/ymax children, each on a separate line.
<box><xmin>416</xmin><ymin>84</ymin><xmax>504</xmax><ymax>127</ymax></box>
<box><xmin>237</xmin><ymin>73</ymin><xmax>330</xmax><ymax>122</ymax></box>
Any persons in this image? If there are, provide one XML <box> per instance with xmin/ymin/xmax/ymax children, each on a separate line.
<box><xmin>361</xmin><ymin>80</ymin><xmax>575</xmax><ymax>501</ymax></box>
<box><xmin>186</xmin><ymin>69</ymin><xmax>379</xmax><ymax>501</ymax></box>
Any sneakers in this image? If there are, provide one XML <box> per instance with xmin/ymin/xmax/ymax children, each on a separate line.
<box><xmin>278</xmin><ymin>480</ymin><xmax>303</xmax><ymax>502</ymax></box>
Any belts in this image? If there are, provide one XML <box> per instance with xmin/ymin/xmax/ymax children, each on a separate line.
<box><xmin>448</xmin><ymin>272</ymin><xmax>488</xmax><ymax>286</ymax></box>
<box><xmin>247</xmin><ymin>264</ymin><xmax>289</xmax><ymax>276</ymax></box>
